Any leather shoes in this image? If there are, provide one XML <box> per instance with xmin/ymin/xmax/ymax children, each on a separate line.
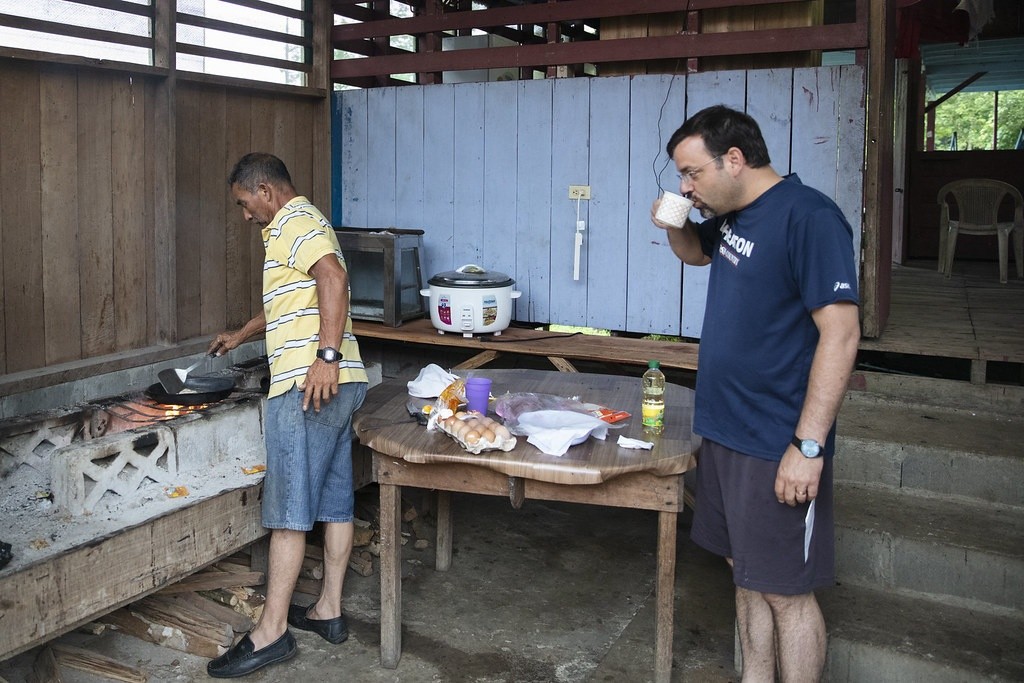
<box><xmin>287</xmin><ymin>603</ymin><xmax>348</xmax><ymax>645</ymax></box>
<box><xmin>207</xmin><ymin>627</ymin><xmax>297</xmax><ymax>678</ymax></box>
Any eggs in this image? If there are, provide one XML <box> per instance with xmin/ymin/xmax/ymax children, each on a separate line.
<box><xmin>444</xmin><ymin>411</ymin><xmax>510</xmax><ymax>444</ymax></box>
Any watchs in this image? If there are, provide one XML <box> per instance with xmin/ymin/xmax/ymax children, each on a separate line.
<box><xmin>316</xmin><ymin>347</ymin><xmax>343</xmax><ymax>363</ymax></box>
<box><xmin>792</xmin><ymin>434</ymin><xmax>824</xmax><ymax>458</ymax></box>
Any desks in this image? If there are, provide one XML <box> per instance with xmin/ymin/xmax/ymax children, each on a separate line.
<box><xmin>349</xmin><ymin>306</ymin><xmax>701</xmax><ymax>373</ymax></box>
<box><xmin>352</xmin><ymin>369</ymin><xmax>704</xmax><ymax>683</ymax></box>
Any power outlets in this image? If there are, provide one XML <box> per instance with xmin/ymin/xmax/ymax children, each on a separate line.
<box><xmin>568</xmin><ymin>185</ymin><xmax>590</xmax><ymax>200</ymax></box>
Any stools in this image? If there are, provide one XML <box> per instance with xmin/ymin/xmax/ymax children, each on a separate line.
<box><xmin>330</xmin><ymin>228</ymin><xmax>430</xmax><ymax>330</ymax></box>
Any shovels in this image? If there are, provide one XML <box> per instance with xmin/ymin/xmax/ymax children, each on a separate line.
<box><xmin>157</xmin><ymin>343</ymin><xmax>224</xmax><ymax>394</ymax></box>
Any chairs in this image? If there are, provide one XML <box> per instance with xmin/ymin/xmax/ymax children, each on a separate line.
<box><xmin>936</xmin><ymin>177</ymin><xmax>1024</xmax><ymax>284</ymax></box>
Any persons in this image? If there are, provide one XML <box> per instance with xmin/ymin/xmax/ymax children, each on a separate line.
<box><xmin>206</xmin><ymin>151</ymin><xmax>369</xmax><ymax>678</ymax></box>
<box><xmin>652</xmin><ymin>104</ymin><xmax>863</xmax><ymax>683</ymax></box>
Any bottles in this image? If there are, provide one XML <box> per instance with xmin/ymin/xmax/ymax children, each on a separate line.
<box><xmin>641</xmin><ymin>360</ymin><xmax>666</xmax><ymax>435</ymax></box>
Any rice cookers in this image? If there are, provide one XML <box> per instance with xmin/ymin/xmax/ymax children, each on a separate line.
<box><xmin>419</xmin><ymin>264</ymin><xmax>522</xmax><ymax>338</ymax></box>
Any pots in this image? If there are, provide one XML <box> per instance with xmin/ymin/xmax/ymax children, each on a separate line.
<box><xmin>146</xmin><ymin>375</ymin><xmax>236</xmax><ymax>405</ymax></box>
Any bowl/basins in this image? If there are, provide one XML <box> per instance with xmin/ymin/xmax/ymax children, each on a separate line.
<box><xmin>572</xmin><ymin>429</ymin><xmax>594</xmax><ymax>445</ymax></box>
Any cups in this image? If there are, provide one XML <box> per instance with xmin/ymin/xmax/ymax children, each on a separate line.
<box><xmin>654</xmin><ymin>191</ymin><xmax>694</xmax><ymax>229</ymax></box>
<box><xmin>466</xmin><ymin>378</ymin><xmax>492</xmax><ymax>417</ymax></box>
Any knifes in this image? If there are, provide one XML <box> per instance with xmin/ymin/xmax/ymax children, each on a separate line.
<box><xmin>405</xmin><ymin>398</ymin><xmax>428</xmax><ymax>426</ymax></box>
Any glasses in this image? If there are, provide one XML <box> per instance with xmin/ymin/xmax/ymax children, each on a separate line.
<box><xmin>677</xmin><ymin>154</ymin><xmax>721</xmax><ymax>185</ymax></box>
